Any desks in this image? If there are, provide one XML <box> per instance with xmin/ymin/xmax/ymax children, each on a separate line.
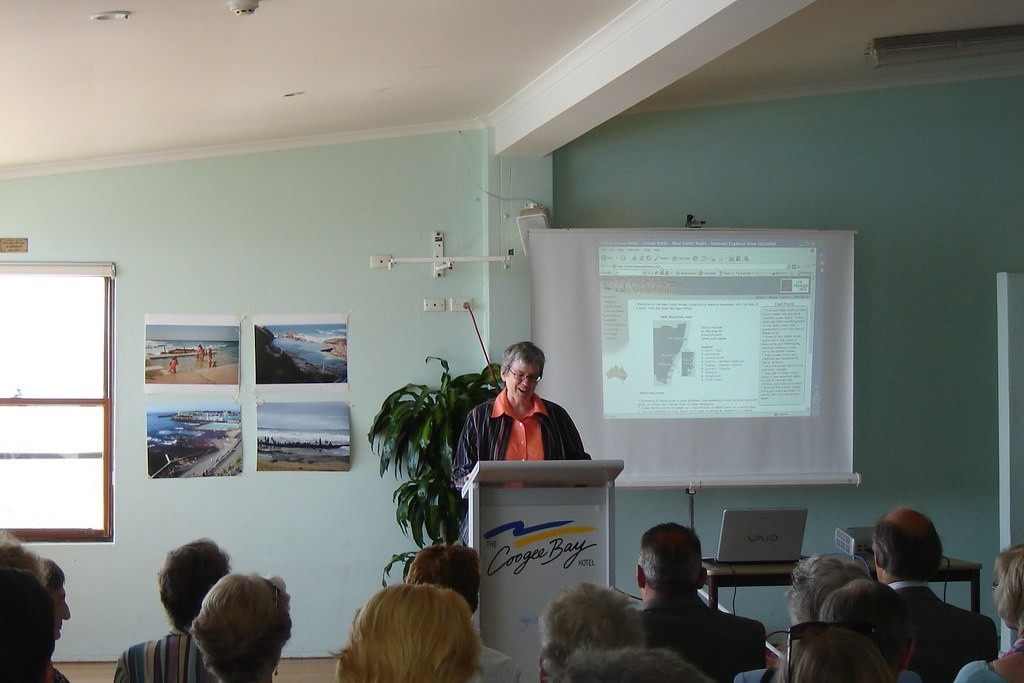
<box><xmin>702</xmin><ymin>558</ymin><xmax>985</xmax><ymax>615</ymax></box>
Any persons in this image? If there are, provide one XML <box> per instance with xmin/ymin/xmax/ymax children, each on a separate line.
<box><xmin>877</xmin><ymin>508</ymin><xmax>998</xmax><ymax>683</ymax></box>
<box><xmin>114</xmin><ymin>537</ymin><xmax>232</xmax><ymax>683</ymax></box>
<box><xmin>406</xmin><ymin>544</ymin><xmax>523</xmax><ymax>683</ymax></box>
<box><xmin>538</xmin><ymin>580</ymin><xmax>712</xmax><ymax>683</ymax></box>
<box><xmin>189</xmin><ymin>571</ymin><xmax>291</xmax><ymax>683</ymax></box>
<box><xmin>329</xmin><ymin>581</ymin><xmax>482</xmax><ymax>683</ymax></box>
<box><xmin>450</xmin><ymin>341</ymin><xmax>592</xmax><ymax>546</ymax></box>
<box><xmin>0</xmin><ymin>529</ymin><xmax>73</xmax><ymax>683</ymax></box>
<box><xmin>633</xmin><ymin>522</ymin><xmax>766</xmax><ymax>683</ymax></box>
<box><xmin>167</xmin><ymin>344</ymin><xmax>218</xmax><ymax>375</ymax></box>
<box><xmin>734</xmin><ymin>550</ymin><xmax>922</xmax><ymax>683</ymax></box>
<box><xmin>954</xmin><ymin>544</ymin><xmax>1024</xmax><ymax>683</ymax></box>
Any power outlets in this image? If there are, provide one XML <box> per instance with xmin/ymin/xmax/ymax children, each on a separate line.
<box><xmin>451</xmin><ymin>298</ymin><xmax>473</xmax><ymax>312</ymax></box>
<box><xmin>424</xmin><ymin>298</ymin><xmax>446</xmax><ymax>312</ymax></box>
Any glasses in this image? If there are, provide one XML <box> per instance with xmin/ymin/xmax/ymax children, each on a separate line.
<box><xmin>258</xmin><ymin>576</ymin><xmax>281</xmax><ymax>615</ymax></box>
<box><xmin>785</xmin><ymin>620</ymin><xmax>887</xmax><ymax>683</ymax></box>
<box><xmin>508</xmin><ymin>366</ymin><xmax>542</xmax><ymax>384</ymax></box>
<box><xmin>992</xmin><ymin>580</ymin><xmax>998</xmax><ymax>590</ymax></box>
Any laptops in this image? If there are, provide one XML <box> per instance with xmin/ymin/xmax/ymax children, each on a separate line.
<box><xmin>702</xmin><ymin>506</ymin><xmax>810</xmax><ymax>563</ymax></box>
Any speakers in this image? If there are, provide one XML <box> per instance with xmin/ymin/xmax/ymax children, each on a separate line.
<box><xmin>516</xmin><ymin>207</ymin><xmax>551</xmax><ymax>257</ymax></box>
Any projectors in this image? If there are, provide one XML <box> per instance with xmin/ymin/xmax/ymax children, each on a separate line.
<box><xmin>834</xmin><ymin>526</ymin><xmax>879</xmax><ymax>558</ymax></box>
